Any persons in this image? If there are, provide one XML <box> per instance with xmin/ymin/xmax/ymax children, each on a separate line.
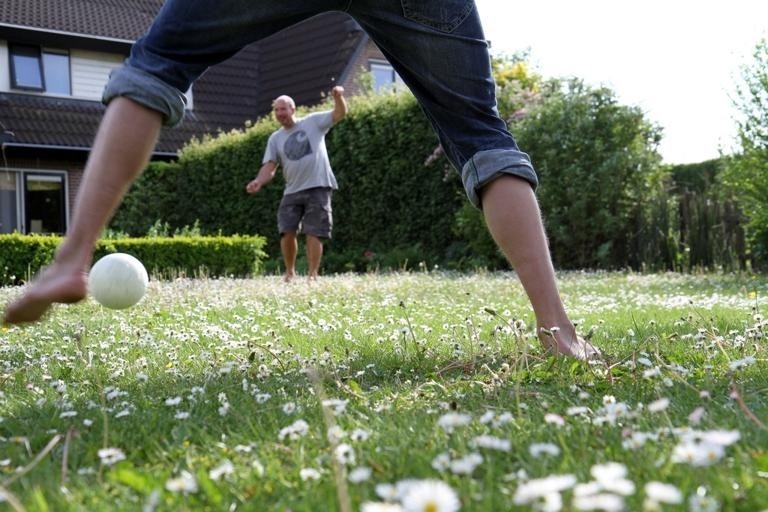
<box><xmin>246</xmin><ymin>86</ymin><xmax>347</xmax><ymax>281</ymax></box>
<box><xmin>3</xmin><ymin>1</ymin><xmax>601</xmax><ymax>364</ymax></box>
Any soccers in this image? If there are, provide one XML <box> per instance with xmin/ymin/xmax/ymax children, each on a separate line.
<box><xmin>88</xmin><ymin>252</ymin><xmax>148</xmax><ymax>310</ymax></box>
